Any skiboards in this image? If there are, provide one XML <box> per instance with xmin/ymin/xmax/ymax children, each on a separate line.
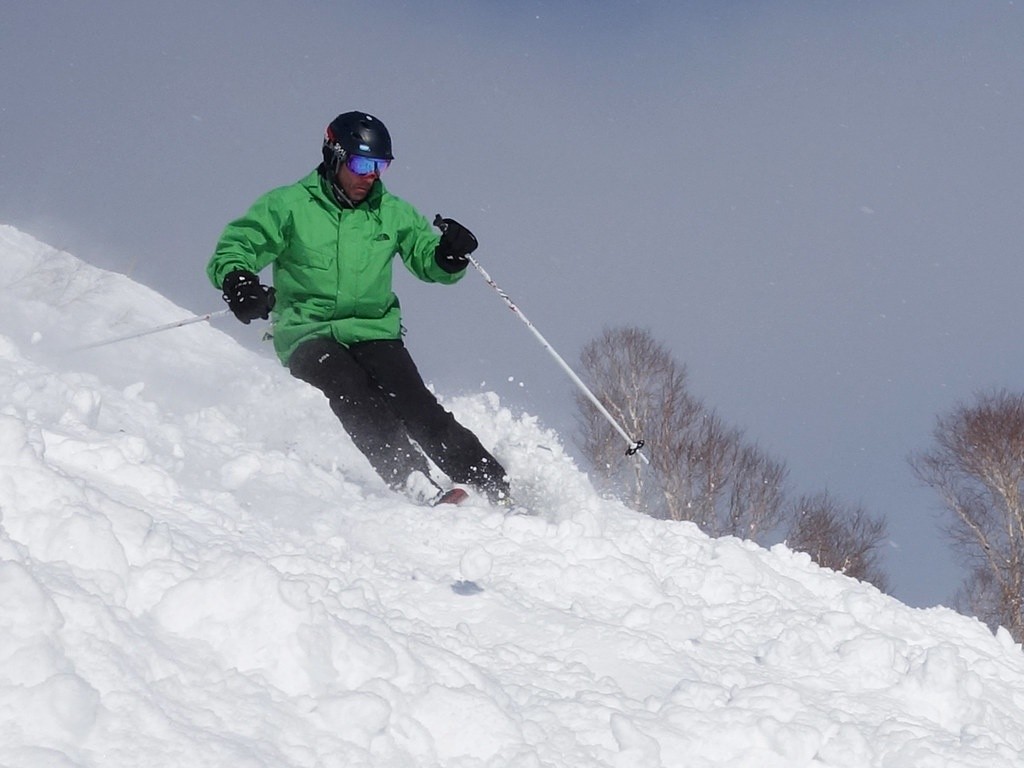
<box><xmin>434</xmin><ymin>485</ymin><xmax>469</xmax><ymax>507</ymax></box>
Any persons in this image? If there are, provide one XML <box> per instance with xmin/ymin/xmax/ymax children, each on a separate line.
<box><xmin>207</xmin><ymin>111</ymin><xmax>538</xmax><ymax>515</ymax></box>
